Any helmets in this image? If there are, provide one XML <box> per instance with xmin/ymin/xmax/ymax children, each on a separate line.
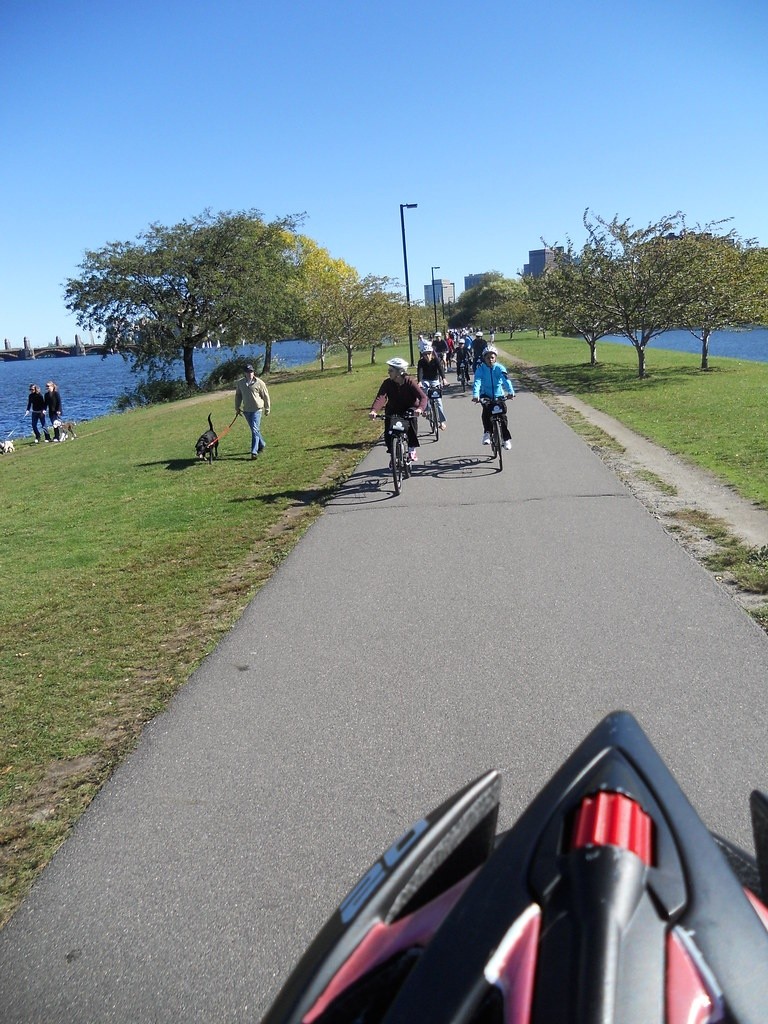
<box><xmin>386</xmin><ymin>358</ymin><xmax>409</xmax><ymax>372</ymax></box>
<box><xmin>482</xmin><ymin>345</ymin><xmax>498</xmax><ymax>358</ymax></box>
<box><xmin>421</xmin><ymin>346</ymin><xmax>433</xmax><ymax>354</ymax></box>
<box><xmin>434</xmin><ymin>329</ymin><xmax>483</xmax><ymax>346</ymax></box>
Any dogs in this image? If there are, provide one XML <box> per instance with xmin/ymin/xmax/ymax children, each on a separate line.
<box><xmin>194</xmin><ymin>411</ymin><xmax>218</xmax><ymax>465</ymax></box>
<box><xmin>52</xmin><ymin>420</ymin><xmax>78</xmax><ymax>442</ymax></box>
<box><xmin>0</xmin><ymin>440</ymin><xmax>15</xmax><ymax>454</ymax></box>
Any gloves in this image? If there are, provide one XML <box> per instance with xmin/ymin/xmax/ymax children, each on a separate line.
<box><xmin>265</xmin><ymin>409</ymin><xmax>270</xmax><ymax>416</ymax></box>
<box><xmin>237</xmin><ymin>409</ymin><xmax>241</xmax><ymax>414</ymax></box>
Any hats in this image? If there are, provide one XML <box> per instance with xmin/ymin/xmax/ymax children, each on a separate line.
<box><xmin>244</xmin><ymin>364</ymin><xmax>255</xmax><ymax>373</ymax></box>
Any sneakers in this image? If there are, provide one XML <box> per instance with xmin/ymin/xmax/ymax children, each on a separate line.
<box><xmin>482</xmin><ymin>431</ymin><xmax>491</xmax><ymax>445</ymax></box>
<box><xmin>409</xmin><ymin>448</ymin><xmax>417</xmax><ymax>462</ymax></box>
<box><xmin>504</xmin><ymin>439</ymin><xmax>511</xmax><ymax>450</ymax></box>
<box><xmin>388</xmin><ymin>457</ymin><xmax>398</xmax><ymax>471</ymax></box>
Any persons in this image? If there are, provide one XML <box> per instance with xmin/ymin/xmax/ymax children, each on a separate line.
<box><xmin>472</xmin><ymin>346</ymin><xmax>514</xmax><ymax>451</ymax></box>
<box><xmin>42</xmin><ymin>381</ymin><xmax>62</xmax><ymax>443</ymax></box>
<box><xmin>234</xmin><ymin>364</ymin><xmax>270</xmax><ymax>459</ymax></box>
<box><xmin>26</xmin><ymin>384</ymin><xmax>51</xmax><ymax>444</ymax></box>
<box><xmin>369</xmin><ymin>358</ymin><xmax>426</xmax><ymax>471</ymax></box>
<box><xmin>416</xmin><ymin>347</ymin><xmax>448</xmax><ymax>430</ymax></box>
<box><xmin>417</xmin><ymin>327</ymin><xmax>487</xmax><ymax>387</ymax></box>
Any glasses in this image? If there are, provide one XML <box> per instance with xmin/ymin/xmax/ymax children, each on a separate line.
<box><xmin>387</xmin><ymin>369</ymin><xmax>393</xmax><ymax>372</ymax></box>
<box><xmin>485</xmin><ymin>356</ymin><xmax>494</xmax><ymax>360</ymax></box>
<box><xmin>46</xmin><ymin>386</ymin><xmax>49</xmax><ymax>387</ymax></box>
<box><xmin>29</xmin><ymin>389</ymin><xmax>34</xmax><ymax>391</ymax></box>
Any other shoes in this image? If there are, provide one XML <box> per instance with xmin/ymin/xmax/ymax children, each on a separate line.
<box><xmin>457</xmin><ymin>375</ymin><xmax>462</xmax><ymax>381</ymax></box>
<box><xmin>467</xmin><ymin>380</ymin><xmax>471</xmax><ymax>386</ymax></box>
<box><xmin>422</xmin><ymin>411</ymin><xmax>427</xmax><ymax>417</ymax></box>
<box><xmin>257</xmin><ymin>445</ymin><xmax>263</xmax><ymax>453</ymax></box>
<box><xmin>440</xmin><ymin>422</ymin><xmax>446</xmax><ymax>430</ymax></box>
<box><xmin>35</xmin><ymin>437</ymin><xmax>40</xmax><ymax>443</ymax></box>
<box><xmin>45</xmin><ymin>437</ymin><xmax>52</xmax><ymax>442</ymax></box>
<box><xmin>53</xmin><ymin>438</ymin><xmax>58</xmax><ymax>442</ymax></box>
<box><xmin>252</xmin><ymin>454</ymin><xmax>256</xmax><ymax>460</ymax></box>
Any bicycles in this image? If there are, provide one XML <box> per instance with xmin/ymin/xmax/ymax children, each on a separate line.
<box><xmin>372</xmin><ymin>410</ymin><xmax>423</xmax><ymax>495</ymax></box>
<box><xmin>416</xmin><ymin>382</ymin><xmax>451</xmax><ymax>441</ymax></box>
<box><xmin>471</xmin><ymin>395</ymin><xmax>517</xmax><ymax>471</ymax></box>
<box><xmin>437</xmin><ymin>350</ymin><xmax>484</xmax><ymax>393</ymax></box>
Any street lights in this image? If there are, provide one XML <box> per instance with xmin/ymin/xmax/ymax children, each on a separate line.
<box><xmin>450</xmin><ymin>282</ymin><xmax>455</xmax><ymax>304</ymax></box>
<box><xmin>441</xmin><ymin>286</ymin><xmax>447</xmax><ymax>319</ymax></box>
<box><xmin>400</xmin><ymin>202</ymin><xmax>418</xmax><ymax>366</ymax></box>
<box><xmin>431</xmin><ymin>266</ymin><xmax>441</xmax><ymax>332</ymax></box>
